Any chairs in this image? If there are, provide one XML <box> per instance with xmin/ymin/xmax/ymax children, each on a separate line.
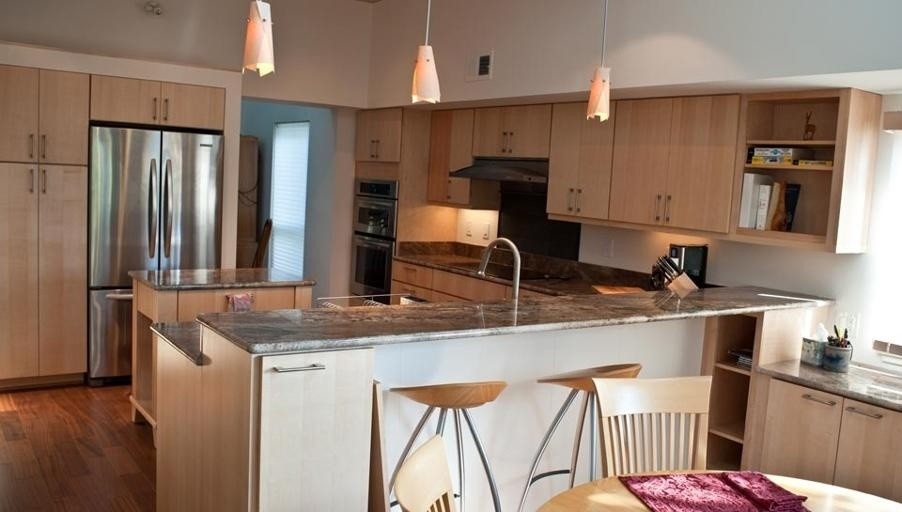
<box><xmin>592</xmin><ymin>376</ymin><xmax>712</xmax><ymax>479</ymax></box>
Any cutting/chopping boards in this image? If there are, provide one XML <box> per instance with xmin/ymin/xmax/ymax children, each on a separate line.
<box><xmin>591</xmin><ymin>285</ymin><xmax>646</xmax><ymax>294</ymax></box>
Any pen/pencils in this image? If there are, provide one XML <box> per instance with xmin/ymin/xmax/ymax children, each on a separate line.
<box><xmin>654</xmin><ymin>254</ymin><xmax>683</xmax><ymax>282</ymax></box>
<box><xmin>827</xmin><ymin>324</ymin><xmax>848</xmax><ymax>349</ymax></box>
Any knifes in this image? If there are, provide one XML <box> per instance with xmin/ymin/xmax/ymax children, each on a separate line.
<box><xmin>655</xmin><ymin>254</ymin><xmax>682</xmax><ymax>282</ymax></box>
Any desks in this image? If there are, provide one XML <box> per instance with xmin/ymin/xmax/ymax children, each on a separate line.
<box><xmin>127</xmin><ymin>268</ymin><xmax>316</xmax><ymax>448</ymax></box>
<box><xmin>534</xmin><ymin>469</ymin><xmax>902</xmax><ymax>511</ymax></box>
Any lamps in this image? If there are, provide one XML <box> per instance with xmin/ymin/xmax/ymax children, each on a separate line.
<box><xmin>411</xmin><ymin>1</ymin><xmax>441</xmax><ymax>106</ymax></box>
<box><xmin>586</xmin><ymin>2</ymin><xmax>613</xmax><ymax>122</ymax></box>
<box><xmin>241</xmin><ymin>1</ymin><xmax>274</xmax><ymax>78</ymax></box>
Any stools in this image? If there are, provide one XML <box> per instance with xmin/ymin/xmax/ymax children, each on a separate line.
<box><xmin>387</xmin><ymin>381</ymin><xmax>508</xmax><ymax>512</ymax></box>
<box><xmin>516</xmin><ymin>364</ymin><xmax>644</xmax><ymax>512</ymax></box>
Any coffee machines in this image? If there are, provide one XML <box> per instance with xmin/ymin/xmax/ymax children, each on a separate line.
<box><xmin>664</xmin><ymin>243</ymin><xmax>707</xmax><ymax>289</ymax></box>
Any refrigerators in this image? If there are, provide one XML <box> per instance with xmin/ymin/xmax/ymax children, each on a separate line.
<box><xmin>86</xmin><ymin>124</ymin><xmax>225</xmax><ymax>387</ymax></box>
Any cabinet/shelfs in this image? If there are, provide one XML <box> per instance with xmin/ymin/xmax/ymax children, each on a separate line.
<box><xmin>732</xmin><ymin>88</ymin><xmax>880</xmax><ymax>254</ymax></box>
<box><xmin>607</xmin><ymin>90</ymin><xmax>746</xmax><ymax>240</ymax></box>
<box><xmin>388</xmin><ymin>256</ymin><xmax>566</xmax><ymax>305</ymax></box>
<box><xmin>471</xmin><ymin>93</ymin><xmax>553</xmax><ymax>159</ymax></box>
<box><xmin>692</xmin><ymin>306</ymin><xmax>828</xmax><ymax>469</ymax></box>
<box><xmin>0</xmin><ymin>162</ymin><xmax>89</xmax><ymax>392</ymax></box>
<box><xmin>760</xmin><ymin>378</ymin><xmax>902</xmax><ymax>504</ymax></box>
<box><xmin>356</xmin><ymin>106</ymin><xmax>460</xmax><ymax>244</ymax></box>
<box><xmin>547</xmin><ymin>86</ymin><xmax>618</xmax><ymax>224</ymax></box>
<box><xmin>0</xmin><ymin>64</ymin><xmax>90</xmax><ymax>165</ymax></box>
<box><xmin>90</xmin><ymin>74</ymin><xmax>226</xmax><ymax>133</ymax></box>
<box><xmin>424</xmin><ymin>101</ymin><xmax>475</xmax><ymax>208</ymax></box>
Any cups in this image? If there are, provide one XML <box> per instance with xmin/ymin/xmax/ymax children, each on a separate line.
<box><xmin>823</xmin><ymin>343</ymin><xmax>851</xmax><ymax>373</ymax></box>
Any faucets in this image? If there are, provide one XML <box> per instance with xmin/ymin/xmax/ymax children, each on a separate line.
<box><xmin>477</xmin><ymin>238</ymin><xmax>522</xmax><ymax>308</ymax></box>
<box><xmin>474</xmin><ymin>309</ymin><xmax>518</xmax><ymax>328</ymax></box>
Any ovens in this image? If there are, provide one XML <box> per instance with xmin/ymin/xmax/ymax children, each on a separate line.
<box><xmin>349</xmin><ymin>178</ymin><xmax>397</xmax><ymax>305</ymax></box>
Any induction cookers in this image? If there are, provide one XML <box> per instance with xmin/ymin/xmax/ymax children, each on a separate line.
<box><xmin>437</xmin><ymin>260</ymin><xmax>568</xmax><ymax>283</ymax></box>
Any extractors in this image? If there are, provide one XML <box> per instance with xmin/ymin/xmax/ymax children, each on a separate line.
<box><xmin>448</xmin><ymin>159</ymin><xmax>547</xmax><ymax>185</ymax></box>
